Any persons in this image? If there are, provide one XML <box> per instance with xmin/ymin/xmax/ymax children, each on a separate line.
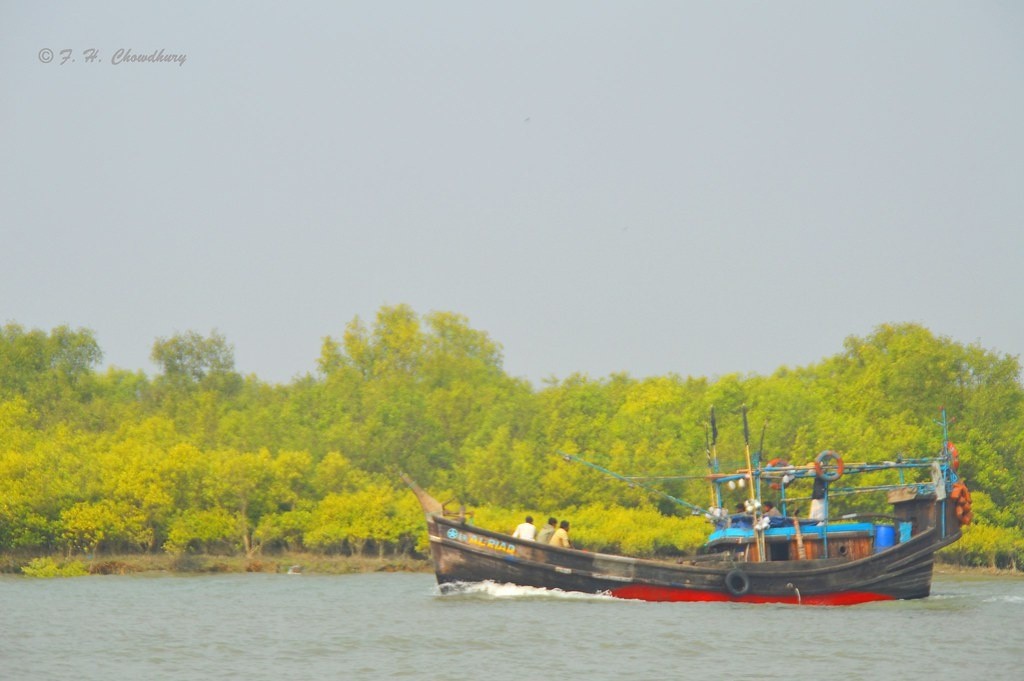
<box><xmin>809</xmin><ymin>476</ymin><xmax>826</xmax><ymax>526</ymax></box>
<box><xmin>549</xmin><ymin>520</ymin><xmax>573</xmax><ymax>549</ymax></box>
<box><xmin>511</xmin><ymin>516</ymin><xmax>537</xmax><ymax>541</ymax></box>
<box><xmin>761</xmin><ymin>501</ymin><xmax>782</xmax><ymax>516</ymax></box>
<box><xmin>735</xmin><ymin>502</ymin><xmax>747</xmax><ymax>515</ymax></box>
<box><xmin>536</xmin><ymin>517</ymin><xmax>557</xmax><ymax>545</ymax></box>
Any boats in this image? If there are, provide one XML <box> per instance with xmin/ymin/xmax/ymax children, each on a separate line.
<box><xmin>426</xmin><ymin>404</ymin><xmax>973</xmax><ymax>606</ymax></box>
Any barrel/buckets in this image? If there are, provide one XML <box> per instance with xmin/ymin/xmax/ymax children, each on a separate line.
<box><xmin>871</xmin><ymin>524</ymin><xmax>896</xmax><ymax>554</ymax></box>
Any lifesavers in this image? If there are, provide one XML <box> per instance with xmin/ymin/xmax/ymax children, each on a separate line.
<box><xmin>762</xmin><ymin>455</ymin><xmax>795</xmax><ymax>491</ymax></box>
<box><xmin>812</xmin><ymin>449</ymin><xmax>846</xmax><ymax>484</ymax></box>
<box><xmin>940</xmin><ymin>441</ymin><xmax>961</xmax><ymax>472</ymax></box>
<box><xmin>721</xmin><ymin>566</ymin><xmax>752</xmax><ymax>598</ymax></box>
